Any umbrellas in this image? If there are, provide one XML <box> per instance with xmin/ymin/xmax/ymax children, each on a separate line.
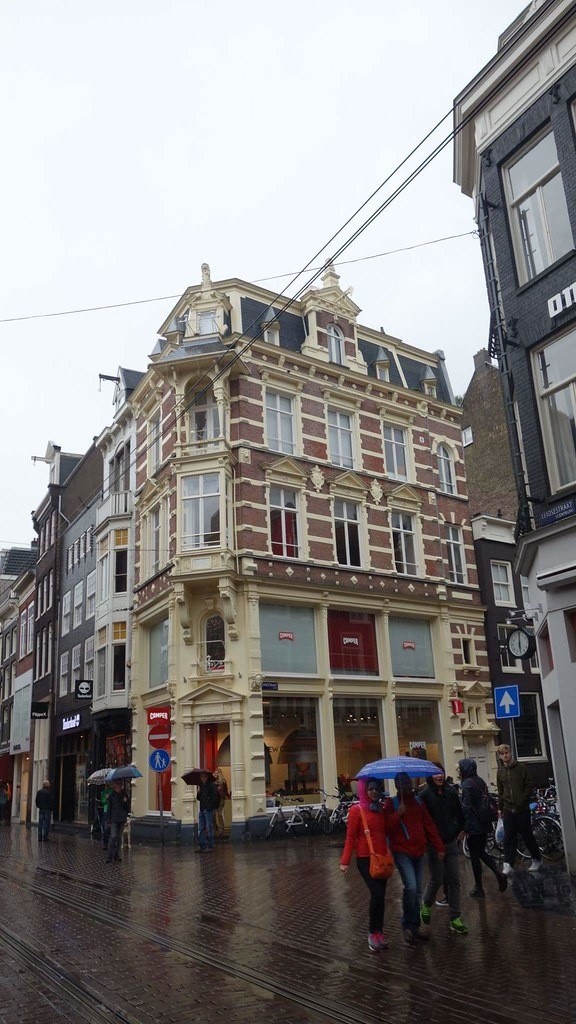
<box><xmin>106</xmin><ymin>766</ymin><xmax>144</xmax><ymax>789</ymax></box>
<box><xmin>354</xmin><ymin>756</ymin><xmax>444</xmax><ymax>802</ymax></box>
<box><xmin>87</xmin><ymin>768</ymin><xmax>114</xmax><ymax>785</ymax></box>
<box><xmin>181</xmin><ymin>768</ymin><xmax>218</xmax><ymax>786</ymax></box>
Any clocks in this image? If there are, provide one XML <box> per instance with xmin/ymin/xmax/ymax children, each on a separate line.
<box><xmin>507</xmin><ymin>628</ymin><xmax>536</xmax><ymax>660</ymax></box>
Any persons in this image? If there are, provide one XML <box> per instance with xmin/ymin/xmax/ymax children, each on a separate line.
<box><xmin>419</xmin><ymin>761</ymin><xmax>469</xmax><ymax>933</ymax></box>
<box><xmin>494</xmin><ymin>745</ymin><xmax>544</xmax><ymax>875</ymax></box>
<box><xmin>212</xmin><ymin>768</ymin><xmax>232</xmax><ymax>837</ymax></box>
<box><xmin>36</xmin><ymin>780</ymin><xmax>55</xmax><ymax>843</ymax></box>
<box><xmin>100</xmin><ymin>780</ymin><xmax>130</xmax><ymax>863</ymax></box>
<box><xmin>338</xmin><ymin>777</ymin><xmax>407</xmax><ymax>954</ymax></box>
<box><xmin>459</xmin><ymin>758</ymin><xmax>508</xmax><ymax>898</ymax></box>
<box><xmin>382</xmin><ymin>773</ymin><xmax>445</xmax><ymax>942</ymax></box>
<box><xmin>193</xmin><ymin>772</ymin><xmax>218</xmax><ymax>853</ymax></box>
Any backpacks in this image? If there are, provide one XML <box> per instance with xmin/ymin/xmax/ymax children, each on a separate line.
<box><xmin>466</xmin><ymin>778</ymin><xmax>499</xmax><ymax>824</ymax></box>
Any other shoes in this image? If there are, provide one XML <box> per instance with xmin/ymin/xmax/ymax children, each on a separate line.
<box><xmin>194</xmin><ymin>849</ymin><xmax>202</xmax><ymax>852</ymax></box>
<box><xmin>470</xmin><ymin>890</ymin><xmax>485</xmax><ymax>901</ymax></box>
<box><xmin>202</xmin><ymin>846</ymin><xmax>213</xmax><ymax>853</ymax></box>
<box><xmin>115</xmin><ymin>857</ymin><xmax>121</xmax><ymax>861</ymax></box>
<box><xmin>404</xmin><ymin>928</ymin><xmax>420</xmax><ymax>943</ymax></box>
<box><xmin>498</xmin><ymin>873</ymin><xmax>508</xmax><ymax>893</ymax></box>
<box><xmin>106</xmin><ymin>861</ymin><xmax>114</xmax><ymax>865</ymax></box>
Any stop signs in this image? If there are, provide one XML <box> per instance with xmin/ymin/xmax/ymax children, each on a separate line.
<box><xmin>147</xmin><ymin>725</ymin><xmax>170</xmax><ymax>749</ymax></box>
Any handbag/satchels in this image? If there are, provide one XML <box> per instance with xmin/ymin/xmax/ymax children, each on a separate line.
<box><xmin>369</xmin><ymin>854</ymin><xmax>394</xmax><ymax>880</ymax></box>
<box><xmin>495</xmin><ymin>817</ymin><xmax>505</xmax><ymax>844</ymax></box>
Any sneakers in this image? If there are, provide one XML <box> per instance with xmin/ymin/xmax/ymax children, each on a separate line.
<box><xmin>435</xmin><ymin>898</ymin><xmax>449</xmax><ymax>906</ymax></box>
<box><xmin>528</xmin><ymin>858</ymin><xmax>543</xmax><ymax>871</ymax></box>
<box><xmin>450</xmin><ymin>917</ymin><xmax>468</xmax><ymax>933</ymax></box>
<box><xmin>368</xmin><ymin>929</ymin><xmax>389</xmax><ymax>952</ymax></box>
<box><xmin>502</xmin><ymin>862</ymin><xmax>515</xmax><ymax>875</ymax></box>
<box><xmin>420</xmin><ymin>899</ymin><xmax>432</xmax><ymax>925</ymax></box>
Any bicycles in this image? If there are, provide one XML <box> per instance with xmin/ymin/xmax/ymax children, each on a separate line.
<box><xmin>89</xmin><ymin>797</ymin><xmax>111</xmax><ymax>835</ymax></box>
<box><xmin>261</xmin><ymin>786</ymin><xmax>360</xmax><ymax>841</ymax></box>
<box><xmin>447</xmin><ymin>776</ymin><xmax>565</xmax><ymax>866</ymax></box>
